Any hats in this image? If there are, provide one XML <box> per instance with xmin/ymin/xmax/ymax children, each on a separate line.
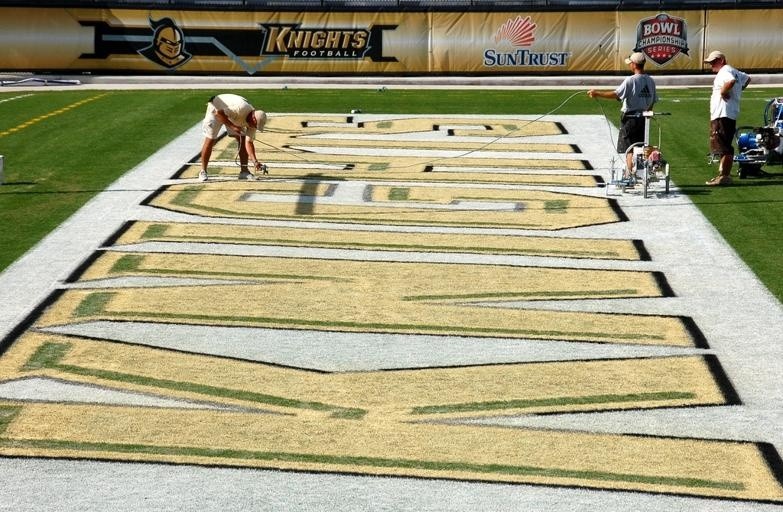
<box><xmin>703</xmin><ymin>51</ymin><xmax>724</xmax><ymax>64</ymax></box>
<box><xmin>625</xmin><ymin>52</ymin><xmax>646</xmax><ymax>64</ymax></box>
<box><xmin>255</xmin><ymin>110</ymin><xmax>266</xmax><ymax>133</ymax></box>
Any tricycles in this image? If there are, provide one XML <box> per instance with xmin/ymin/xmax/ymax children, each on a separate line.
<box><xmin>609</xmin><ymin>111</ymin><xmax>672</xmax><ymax>197</ymax></box>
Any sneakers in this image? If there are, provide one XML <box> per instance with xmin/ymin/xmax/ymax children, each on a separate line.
<box><xmin>239</xmin><ymin>171</ymin><xmax>259</xmax><ymax>179</ymax></box>
<box><xmin>705</xmin><ymin>176</ymin><xmax>728</xmax><ymax>186</ymax></box>
<box><xmin>199</xmin><ymin>170</ymin><xmax>207</xmax><ymax>181</ymax></box>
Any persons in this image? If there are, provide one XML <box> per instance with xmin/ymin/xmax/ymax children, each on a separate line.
<box><xmin>701</xmin><ymin>50</ymin><xmax>751</xmax><ymax>188</ymax></box>
<box><xmin>586</xmin><ymin>52</ymin><xmax>656</xmax><ymax>188</ymax></box>
<box><xmin>198</xmin><ymin>94</ymin><xmax>269</xmax><ymax>182</ymax></box>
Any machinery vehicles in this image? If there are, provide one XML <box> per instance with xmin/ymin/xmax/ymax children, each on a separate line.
<box><xmin>735</xmin><ymin>96</ymin><xmax>782</xmax><ymax>179</ymax></box>
